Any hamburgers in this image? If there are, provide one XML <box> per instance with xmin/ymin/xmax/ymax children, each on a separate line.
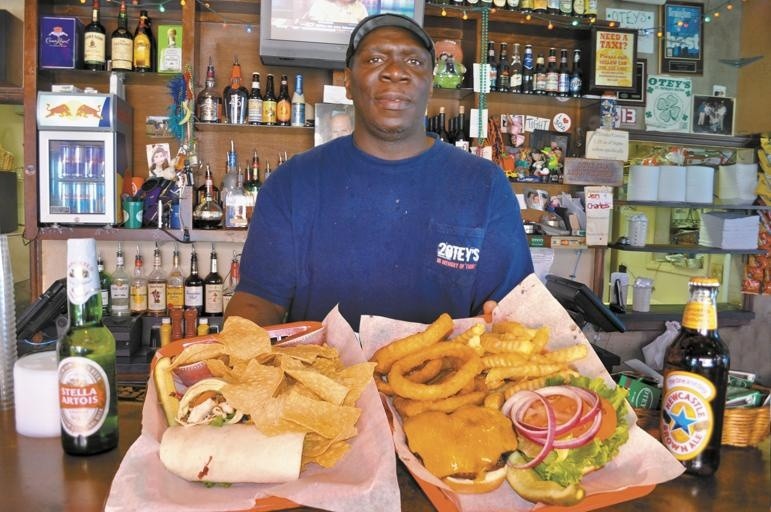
<box><xmin>513</xmin><ymin>374</ymin><xmax>630</xmax><ymax>488</ymax></box>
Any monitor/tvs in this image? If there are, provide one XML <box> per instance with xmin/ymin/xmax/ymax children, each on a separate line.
<box><xmin>544</xmin><ymin>274</ymin><xmax>626</xmax><ymax>333</ymax></box>
<box><xmin>259</xmin><ymin>0</ymin><xmax>425</xmax><ymax>70</ymax></box>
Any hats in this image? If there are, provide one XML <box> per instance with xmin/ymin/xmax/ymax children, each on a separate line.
<box><xmin>347</xmin><ymin>15</ymin><xmax>435</xmax><ymax>70</ymax></box>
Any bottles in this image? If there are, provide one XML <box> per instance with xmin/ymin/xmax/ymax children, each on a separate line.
<box><xmin>547</xmin><ymin>48</ymin><xmax>559</xmax><ymax>96</ymax></box>
<box><xmin>570</xmin><ymin>49</ymin><xmax>582</xmax><ymax>97</ymax></box>
<box><xmin>510</xmin><ymin>43</ymin><xmax>523</xmax><ymax>93</ymax></box>
<box><xmin>56</xmin><ymin>238</ymin><xmax>119</xmax><ymax>457</ymax></box>
<box><xmin>291</xmin><ymin>72</ymin><xmax>305</xmax><ymax>126</ymax></box>
<box><xmin>147</xmin><ymin>248</ymin><xmax>166</xmax><ymax>311</ymax></box>
<box><xmin>481</xmin><ymin>0</ymin><xmax>493</xmax><ymax>8</ymax></box>
<box><xmin>192</xmin><ymin>140</ymin><xmax>289</xmax><ymax>230</ymax></box>
<box><xmin>197</xmin><ymin>65</ymin><xmax>222</xmax><ymax>122</ymax></box>
<box><xmin>453</xmin><ymin>0</ymin><xmax>463</xmax><ymax>5</ymax></box>
<box><xmin>229</xmin><ymin>258</ymin><xmax>239</xmax><ymax>288</ymax></box>
<box><xmin>81</xmin><ymin>0</ymin><xmax>107</xmax><ymax>70</ymax></box>
<box><xmin>130</xmin><ymin>255</ymin><xmax>147</xmax><ymax>313</ymax></box>
<box><xmin>203</xmin><ymin>251</ymin><xmax>223</xmax><ymax>316</ymax></box>
<box><xmin>488</xmin><ymin>41</ymin><xmax>498</xmax><ymax>92</ymax></box>
<box><xmin>559</xmin><ymin>49</ymin><xmax>570</xmax><ymax>97</ymax></box>
<box><xmin>111</xmin><ymin>251</ymin><xmax>130</xmax><ymax>316</ymax></box>
<box><xmin>184</xmin><ymin>161</ymin><xmax>194</xmax><ymax>186</ymax></box>
<box><xmin>161</xmin><ymin>317</ymin><xmax>172</xmax><ymax>347</ymax></box>
<box><xmin>534</xmin><ymin>0</ymin><xmax>548</xmax><ymax>14</ymax></box>
<box><xmin>157</xmin><ymin>196</ymin><xmax>171</xmax><ymax>228</ymax></box>
<box><xmin>277</xmin><ymin>75</ymin><xmax>291</xmax><ymax>125</ymax></box>
<box><xmin>520</xmin><ymin>0</ymin><xmax>533</xmax><ymax>13</ymax></box>
<box><xmin>223</xmin><ymin>64</ymin><xmax>249</xmax><ymax>124</ymax></box>
<box><xmin>133</xmin><ymin>10</ymin><xmax>155</xmax><ymax>72</ymax></box>
<box><xmin>498</xmin><ymin>43</ymin><xmax>510</xmax><ymax>92</ymax></box>
<box><xmin>507</xmin><ymin>0</ymin><xmax>520</xmax><ymax>11</ymax></box>
<box><xmin>189</xmin><ymin>137</ymin><xmax>201</xmax><ymax>168</ymax></box>
<box><xmin>659</xmin><ymin>278</ymin><xmax>731</xmax><ymax>477</ymax></box>
<box><xmin>429</xmin><ymin>104</ymin><xmax>473</xmax><ymax>154</ymax></box>
<box><xmin>534</xmin><ymin>54</ymin><xmax>546</xmax><ymax>94</ymax></box>
<box><xmin>584</xmin><ymin>0</ymin><xmax>597</xmax><ymax>18</ymax></box>
<box><xmin>523</xmin><ymin>45</ymin><xmax>533</xmax><ymax>94</ymax></box>
<box><xmin>247</xmin><ymin>72</ymin><xmax>263</xmax><ymax>125</ymax></box>
<box><xmin>647</xmin><ymin>474</ymin><xmax>718</xmax><ymax>511</ymax></box>
<box><xmin>548</xmin><ymin>0</ymin><xmax>559</xmax><ymax>14</ymax></box>
<box><xmin>111</xmin><ymin>0</ymin><xmax>133</xmax><ymax>71</ymax></box>
<box><xmin>559</xmin><ymin>0</ymin><xmax>572</xmax><ymax>15</ymax></box>
<box><xmin>167</xmin><ymin>251</ymin><xmax>185</xmax><ymax>309</ymax></box>
<box><xmin>263</xmin><ymin>74</ymin><xmax>277</xmax><ymax>125</ymax></box>
<box><xmin>97</xmin><ymin>257</ymin><xmax>110</xmax><ymax>316</ymax></box>
<box><xmin>198</xmin><ymin>318</ymin><xmax>209</xmax><ymax>335</ymax></box>
<box><xmin>184</xmin><ymin>253</ymin><xmax>204</xmax><ymax>316</ymax></box>
<box><xmin>466</xmin><ymin>0</ymin><xmax>478</xmax><ymax>7</ymax></box>
<box><xmin>573</xmin><ymin>0</ymin><xmax>584</xmax><ymax>17</ymax></box>
<box><xmin>493</xmin><ymin>0</ymin><xmax>507</xmax><ymax>9</ymax></box>
<box><xmin>174</xmin><ymin>146</ymin><xmax>187</xmax><ymax>170</ymax></box>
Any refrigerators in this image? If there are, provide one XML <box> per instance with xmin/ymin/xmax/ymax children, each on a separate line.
<box><xmin>34</xmin><ymin>91</ymin><xmax>131</xmax><ymax>229</ymax></box>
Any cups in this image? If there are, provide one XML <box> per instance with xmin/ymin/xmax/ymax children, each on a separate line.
<box><xmin>0</xmin><ymin>233</ymin><xmax>18</xmax><ymax>411</ymax></box>
<box><xmin>633</xmin><ymin>288</ymin><xmax>651</xmax><ymax>312</ymax></box>
<box><xmin>629</xmin><ymin>220</ymin><xmax>647</xmax><ymax>247</ymax></box>
<box><xmin>123</xmin><ymin>201</ymin><xmax>144</xmax><ymax>229</ymax></box>
<box><xmin>13</xmin><ymin>350</ymin><xmax>61</xmax><ymax>437</ymax></box>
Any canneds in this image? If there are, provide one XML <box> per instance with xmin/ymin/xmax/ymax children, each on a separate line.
<box><xmin>49</xmin><ymin>144</ymin><xmax>113</xmax><ymax>218</ymax></box>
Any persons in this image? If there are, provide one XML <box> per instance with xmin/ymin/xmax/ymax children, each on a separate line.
<box><xmin>309</xmin><ymin>0</ymin><xmax>369</xmax><ymax>28</ymax></box>
<box><xmin>548</xmin><ymin>143</ymin><xmax>563</xmax><ymax>174</ymax></box>
<box><xmin>515</xmin><ymin>149</ymin><xmax>531</xmax><ymax>176</ymax></box>
<box><xmin>531</xmin><ymin>152</ymin><xmax>549</xmax><ymax>177</ymax></box>
<box><xmin>698</xmin><ymin>99</ymin><xmax>726</xmax><ymax>133</ymax></box>
<box><xmin>330</xmin><ymin>111</ymin><xmax>353</xmax><ymax>141</ymax></box>
<box><xmin>150</xmin><ymin>148</ymin><xmax>169</xmax><ymax>177</ymax></box>
<box><xmin>223</xmin><ymin>12</ymin><xmax>536</xmax><ymax>329</ymax></box>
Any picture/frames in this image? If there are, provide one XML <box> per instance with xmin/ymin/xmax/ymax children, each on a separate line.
<box><xmin>586</xmin><ymin>24</ymin><xmax>638</xmax><ymax>94</ymax></box>
<box><xmin>689</xmin><ymin>93</ymin><xmax>737</xmax><ymax>138</ymax></box>
<box><xmin>616</xmin><ymin>58</ymin><xmax>648</xmax><ymax>108</ymax></box>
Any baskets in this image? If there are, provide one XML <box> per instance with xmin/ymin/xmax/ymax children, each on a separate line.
<box><xmin>610</xmin><ymin>371</ymin><xmax>771</xmax><ymax>448</ymax></box>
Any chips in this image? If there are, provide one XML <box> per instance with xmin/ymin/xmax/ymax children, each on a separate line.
<box><xmin>167</xmin><ymin>317</ymin><xmax>378</xmax><ymax>471</ymax></box>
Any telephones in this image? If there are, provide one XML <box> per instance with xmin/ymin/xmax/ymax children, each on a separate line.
<box><xmin>610</xmin><ymin>279</ymin><xmax>626</xmax><ymax>312</ymax></box>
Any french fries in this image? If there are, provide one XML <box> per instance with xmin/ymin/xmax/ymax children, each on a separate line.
<box><xmin>451</xmin><ymin>317</ymin><xmax>586</xmax><ymax>411</ymax></box>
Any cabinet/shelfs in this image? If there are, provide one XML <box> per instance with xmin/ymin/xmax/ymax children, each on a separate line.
<box><xmin>22</xmin><ymin>0</ymin><xmax>621</xmax><ymax>251</ymax></box>
<box><xmin>593</xmin><ymin>127</ymin><xmax>771</xmax><ymax>333</ymax></box>
